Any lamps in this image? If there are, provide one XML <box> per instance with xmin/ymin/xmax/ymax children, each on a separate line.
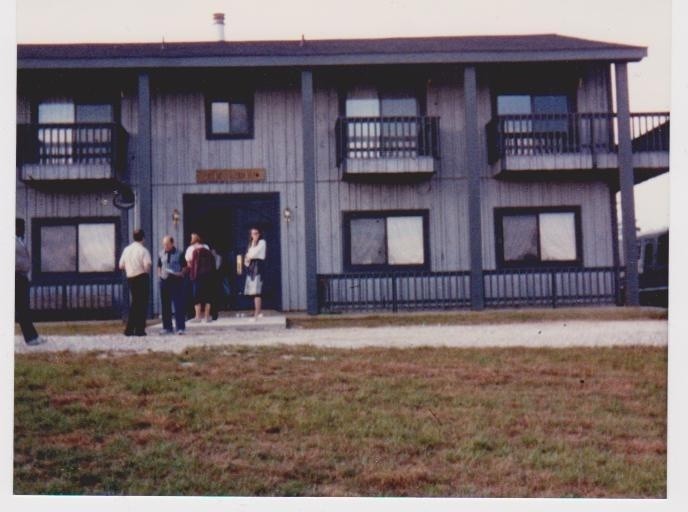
<box><xmin>172</xmin><ymin>208</ymin><xmax>180</xmax><ymax>225</ymax></box>
<box><xmin>284</xmin><ymin>208</ymin><xmax>294</xmax><ymax>226</ymax></box>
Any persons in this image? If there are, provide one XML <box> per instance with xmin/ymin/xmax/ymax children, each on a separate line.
<box><xmin>16</xmin><ymin>218</ymin><xmax>47</xmax><ymax>346</ymax></box>
<box><xmin>157</xmin><ymin>235</ymin><xmax>187</xmax><ymax>335</ymax></box>
<box><xmin>184</xmin><ymin>232</ymin><xmax>221</xmax><ymax>323</ymax></box>
<box><xmin>118</xmin><ymin>228</ymin><xmax>152</xmax><ymax>337</ymax></box>
<box><xmin>242</xmin><ymin>226</ymin><xmax>266</xmax><ymax>322</ymax></box>
